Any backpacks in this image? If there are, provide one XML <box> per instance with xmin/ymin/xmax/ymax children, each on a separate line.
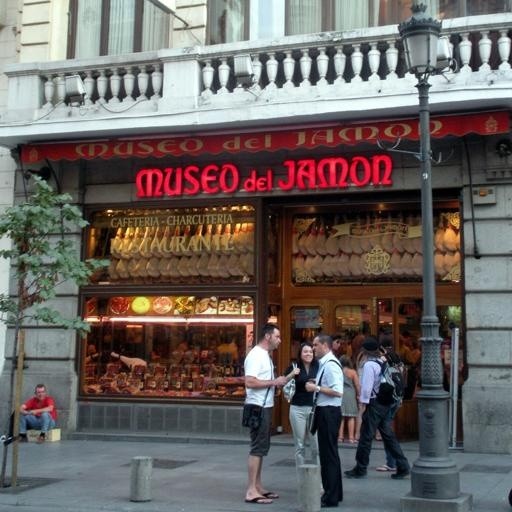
<box><xmin>368</xmin><ymin>358</ymin><xmax>404</xmax><ymax>405</ymax></box>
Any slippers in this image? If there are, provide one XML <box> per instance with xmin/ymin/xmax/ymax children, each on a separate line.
<box><xmin>245</xmin><ymin>492</ymin><xmax>279</xmax><ymax>503</ymax></box>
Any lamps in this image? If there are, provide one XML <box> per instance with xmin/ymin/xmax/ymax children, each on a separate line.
<box><xmin>64</xmin><ymin>74</ymin><xmax>88</xmax><ymax>108</ymax></box>
<box><xmin>234</xmin><ymin>54</ymin><xmax>258</xmax><ymax>95</ymax></box>
<box><xmin>435</xmin><ymin>35</ymin><xmax>454</xmax><ymax>76</ymax></box>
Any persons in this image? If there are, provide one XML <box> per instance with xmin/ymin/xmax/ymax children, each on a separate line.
<box><xmin>18</xmin><ymin>383</ymin><xmax>59</xmax><ymax>445</ymax></box>
<box><xmin>337</xmin><ymin>353</ymin><xmax>361</xmax><ymax>444</ymax></box>
<box><xmin>304</xmin><ymin>333</ymin><xmax>344</xmax><ymax>508</ymax></box>
<box><xmin>350</xmin><ymin>332</ymin><xmax>409</xmax><ymax>473</ymax></box>
<box><xmin>283</xmin><ymin>342</ymin><xmax>321</xmax><ymax>468</ymax></box>
<box><xmin>328</xmin><ymin>332</ymin><xmax>344</xmax><ymax>351</ymax></box>
<box><xmin>242</xmin><ymin>323</ymin><xmax>281</xmax><ymax>505</ymax></box>
<box><xmin>343</xmin><ymin>336</ymin><xmax>413</xmax><ymax>481</ymax></box>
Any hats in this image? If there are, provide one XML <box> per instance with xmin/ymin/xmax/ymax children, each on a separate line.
<box><xmin>362</xmin><ymin>338</ymin><xmax>377</xmax><ymax>351</ymax></box>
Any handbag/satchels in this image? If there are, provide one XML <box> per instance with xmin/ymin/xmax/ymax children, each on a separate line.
<box><xmin>308</xmin><ymin>404</ymin><xmax>318</xmax><ymax>436</ymax></box>
<box><xmin>282</xmin><ymin>378</ymin><xmax>296</xmax><ymax>402</ymax></box>
<box><xmin>242</xmin><ymin>403</ymin><xmax>264</xmax><ymax>430</ymax></box>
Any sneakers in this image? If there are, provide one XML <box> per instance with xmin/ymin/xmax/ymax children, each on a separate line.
<box><xmin>17</xmin><ymin>436</ymin><xmax>28</xmax><ymax>442</ymax></box>
<box><xmin>338</xmin><ymin>438</ymin><xmax>359</xmax><ymax>443</ymax></box>
<box><xmin>377</xmin><ymin>464</ymin><xmax>397</xmax><ymax>471</ymax></box>
<box><xmin>36</xmin><ymin>436</ymin><xmax>44</xmax><ymax>443</ymax></box>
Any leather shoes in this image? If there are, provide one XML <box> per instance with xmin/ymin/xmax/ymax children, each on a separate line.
<box><xmin>391</xmin><ymin>469</ymin><xmax>408</xmax><ymax>479</ymax></box>
<box><xmin>344</xmin><ymin>470</ymin><xmax>366</xmax><ymax>477</ymax></box>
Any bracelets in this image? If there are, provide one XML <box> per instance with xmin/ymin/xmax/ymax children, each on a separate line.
<box><xmin>316</xmin><ymin>385</ymin><xmax>320</xmax><ymax>392</ymax></box>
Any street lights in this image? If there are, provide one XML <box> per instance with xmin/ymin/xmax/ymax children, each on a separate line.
<box><xmin>393</xmin><ymin>1</ymin><xmax>480</xmax><ymax>509</ymax></box>
